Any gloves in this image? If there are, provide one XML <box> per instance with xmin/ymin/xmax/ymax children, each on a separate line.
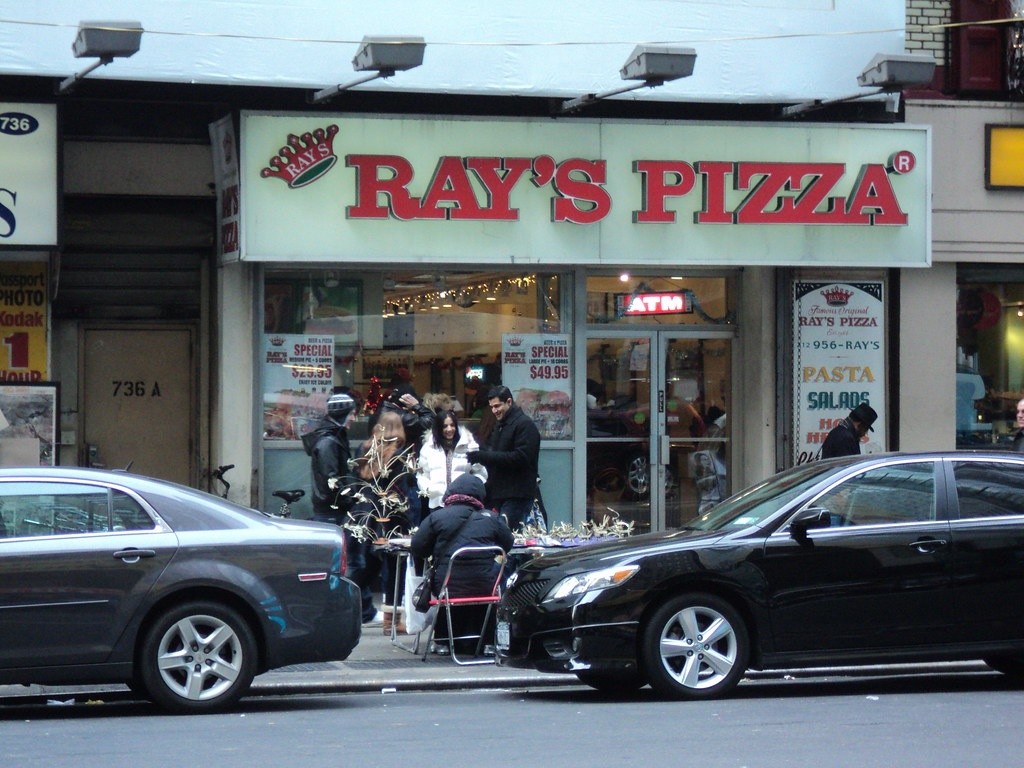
<box><xmin>466</xmin><ymin>452</ymin><xmax>481</xmax><ymax>465</ymax></box>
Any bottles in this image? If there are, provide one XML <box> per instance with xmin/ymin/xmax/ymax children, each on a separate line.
<box><xmin>364</xmin><ymin>358</ymin><xmax>407</xmax><ymax>379</ymax></box>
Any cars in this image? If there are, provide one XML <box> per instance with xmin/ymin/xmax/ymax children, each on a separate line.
<box><xmin>1</xmin><ymin>467</ymin><xmax>368</xmax><ymax>717</ymax></box>
<box><xmin>499</xmin><ymin>450</ymin><xmax>1024</xmax><ymax>702</ymax></box>
<box><xmin>587</xmin><ymin>390</ymin><xmax>726</xmax><ymax>499</ymax></box>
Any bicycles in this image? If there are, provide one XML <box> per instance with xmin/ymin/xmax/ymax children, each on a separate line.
<box><xmin>206</xmin><ymin>464</ymin><xmax>306</xmax><ymax>519</ymax></box>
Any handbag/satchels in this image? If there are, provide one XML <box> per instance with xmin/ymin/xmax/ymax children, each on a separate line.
<box><xmin>412</xmin><ymin>568</ymin><xmax>432</xmax><ymax>613</ymax></box>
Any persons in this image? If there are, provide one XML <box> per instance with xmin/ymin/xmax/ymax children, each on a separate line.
<box><xmin>410</xmin><ymin>472</ymin><xmax>516</xmax><ymax>658</ymax></box>
<box><xmin>1012</xmin><ymin>398</ymin><xmax>1024</xmax><ymax>451</ymax></box>
<box><xmin>301</xmin><ymin>383</ymin><xmax>480</xmax><ymax>634</ymax></box>
<box><xmin>465</xmin><ymin>385</ymin><xmax>541</xmax><ymax>530</ymax></box>
<box><xmin>821</xmin><ymin>401</ymin><xmax>878</xmax><ymax>460</ymax></box>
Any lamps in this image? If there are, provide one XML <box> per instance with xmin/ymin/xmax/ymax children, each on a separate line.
<box><xmin>58</xmin><ymin>22</ymin><xmax>145</xmax><ymax>92</ymax></box>
<box><xmin>313</xmin><ymin>35</ymin><xmax>427</xmax><ymax>103</ymax></box>
<box><xmin>782</xmin><ymin>52</ymin><xmax>937</xmax><ymax>118</ymax></box>
<box><xmin>560</xmin><ymin>45</ymin><xmax>697</xmax><ymax>112</ymax></box>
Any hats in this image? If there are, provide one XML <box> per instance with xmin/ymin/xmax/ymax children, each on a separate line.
<box><xmin>328</xmin><ymin>394</ymin><xmax>355</xmax><ymax>427</ymax></box>
<box><xmin>394</xmin><ymin>384</ymin><xmax>421</xmax><ymax>402</ymax></box>
<box><xmin>848</xmin><ymin>402</ymin><xmax>877</xmax><ymax>434</ymax></box>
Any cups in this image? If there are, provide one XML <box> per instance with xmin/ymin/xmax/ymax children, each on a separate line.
<box><xmin>291</xmin><ymin>417</ymin><xmax>308</xmax><ymax>439</ymax></box>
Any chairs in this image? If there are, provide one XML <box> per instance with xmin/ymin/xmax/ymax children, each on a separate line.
<box><xmin>420</xmin><ymin>546</ymin><xmax>506</xmax><ymax>664</ymax></box>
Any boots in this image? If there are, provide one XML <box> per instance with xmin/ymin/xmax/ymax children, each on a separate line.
<box><xmin>381</xmin><ymin>605</ymin><xmax>408</xmax><ymax>636</ymax></box>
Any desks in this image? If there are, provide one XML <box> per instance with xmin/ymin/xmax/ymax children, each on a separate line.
<box><xmin>384</xmin><ymin>537</ymin><xmax>628</xmax><ymax>653</ymax></box>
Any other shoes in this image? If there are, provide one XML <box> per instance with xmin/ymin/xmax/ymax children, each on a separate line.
<box><xmin>361</xmin><ymin>612</ymin><xmax>384</xmax><ymax>627</ymax></box>
<box><xmin>484</xmin><ymin>645</ymin><xmax>498</xmax><ymax>657</ymax></box>
<box><xmin>430</xmin><ymin>640</ymin><xmax>450</xmax><ymax>656</ymax></box>
